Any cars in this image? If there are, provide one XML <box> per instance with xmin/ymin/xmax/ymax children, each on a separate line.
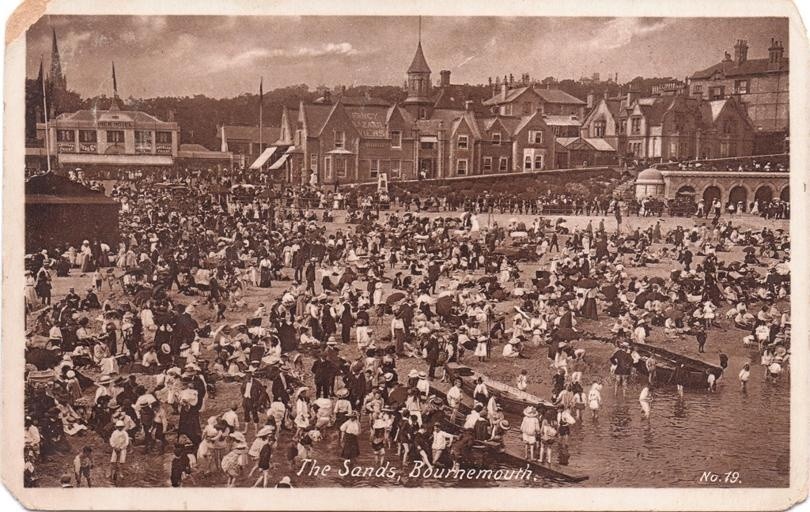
<box><xmin>229</xmin><ymin>184</ymin><xmax>258</xmax><ymax>194</ymax></box>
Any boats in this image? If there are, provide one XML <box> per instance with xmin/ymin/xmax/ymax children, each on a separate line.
<box><xmin>444</xmin><ymin>358</ymin><xmax>559</xmax><ymax>418</ymax></box>
<box><xmin>396</xmin><ymin>381</ymin><xmax>505</xmax><ymax>471</ymax></box>
<box><xmin>617</xmin><ymin>339</ymin><xmax>721</xmax><ymax>390</ymax></box>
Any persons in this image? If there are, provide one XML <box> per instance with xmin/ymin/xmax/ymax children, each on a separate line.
<box><xmin>26</xmin><ymin>162</ymin><xmax>789</xmax><ymax>486</ymax></box>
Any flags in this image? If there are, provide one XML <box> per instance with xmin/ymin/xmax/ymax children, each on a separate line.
<box><xmin>112</xmin><ymin>59</ymin><xmax>119</xmax><ymax>92</ymax></box>
<box><xmin>33</xmin><ymin>59</ymin><xmax>46</xmax><ymax>114</ymax></box>
<box><xmin>259</xmin><ymin>81</ymin><xmax>265</xmax><ymax>106</ymax></box>
<box><xmin>51</xmin><ymin>27</ymin><xmax>65</xmax><ymax>95</ymax></box>
